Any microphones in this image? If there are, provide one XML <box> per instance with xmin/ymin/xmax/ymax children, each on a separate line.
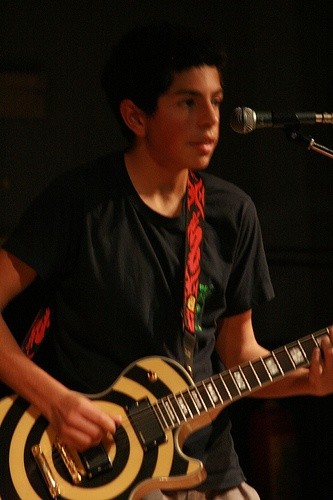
<box><xmin>229</xmin><ymin>106</ymin><xmax>333</xmax><ymax>134</ymax></box>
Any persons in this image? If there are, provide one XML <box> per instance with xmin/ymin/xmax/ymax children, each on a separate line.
<box><xmin>0</xmin><ymin>33</ymin><xmax>333</xmax><ymax>500</ymax></box>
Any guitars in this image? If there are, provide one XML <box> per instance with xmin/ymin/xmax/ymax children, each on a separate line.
<box><xmin>0</xmin><ymin>327</ymin><xmax>333</xmax><ymax>499</ymax></box>
